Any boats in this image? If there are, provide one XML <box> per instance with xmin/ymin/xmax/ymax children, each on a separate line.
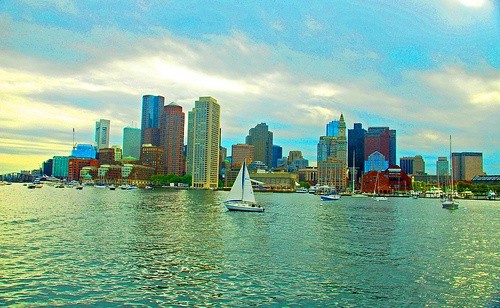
<box><xmin>371</xmin><ymin>196</ymin><xmax>388</xmax><ymax>201</ymax></box>
<box><xmin>0</xmin><ymin>181</ymin><xmax>11</xmax><ymax>185</ymax></box>
<box><xmin>94</xmin><ymin>183</ymin><xmax>153</xmax><ymax>190</ymax></box>
<box><xmin>23</xmin><ymin>179</ymin><xmax>86</xmax><ymax>190</ymax></box>
<box><xmin>320</xmin><ymin>192</ymin><xmax>341</xmax><ymax>200</ymax></box>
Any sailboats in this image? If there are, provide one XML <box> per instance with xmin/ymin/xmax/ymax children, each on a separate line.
<box><xmin>223</xmin><ymin>158</ymin><xmax>264</xmax><ymax>212</ymax></box>
<box><xmin>441</xmin><ymin>134</ymin><xmax>459</xmax><ymax>209</ymax></box>
